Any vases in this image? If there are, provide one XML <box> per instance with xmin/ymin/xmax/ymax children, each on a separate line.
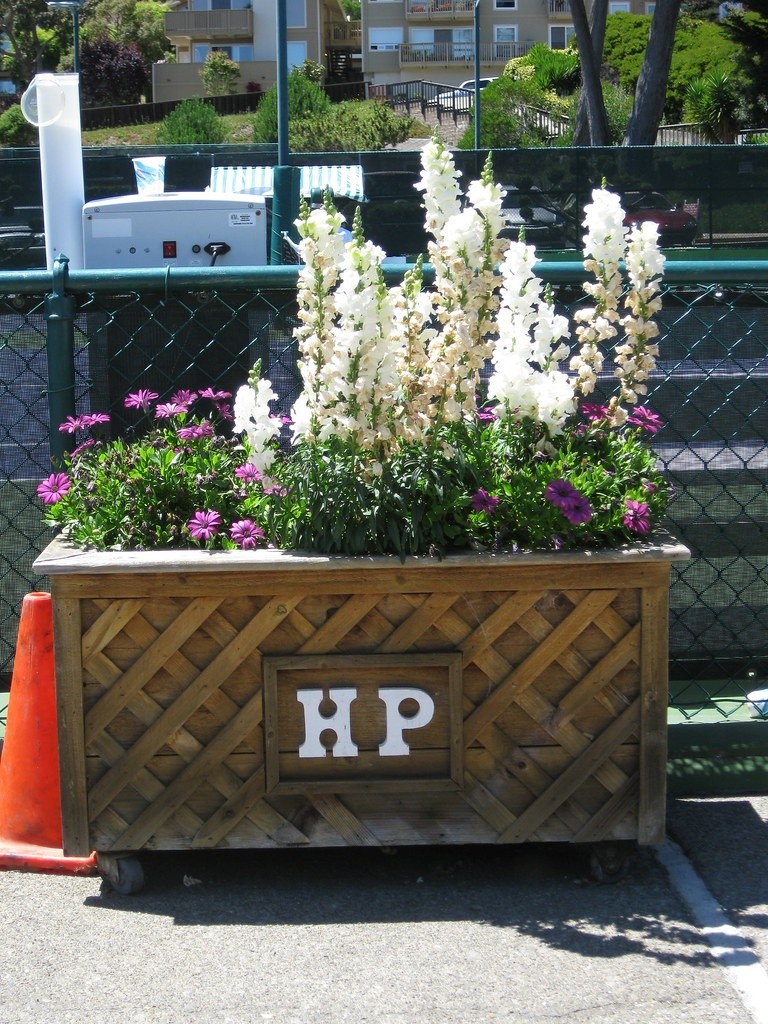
<box><xmin>32</xmin><ymin>521</ymin><xmax>692</xmax><ymax>890</ymax></box>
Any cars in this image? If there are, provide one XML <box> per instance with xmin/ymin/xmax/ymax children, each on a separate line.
<box><xmin>616</xmin><ymin>190</ymin><xmax>699</xmax><ymax>247</ymax></box>
<box><xmin>0</xmin><ymin>222</ymin><xmax>48</xmax><ymax>313</ymax></box>
<box><xmin>337</xmin><ymin>171</ymin><xmax>433</xmax><ymax>257</ymax></box>
<box><xmin>494</xmin><ymin>183</ymin><xmax>564</xmax><ymax>251</ymax></box>
<box><xmin>429</xmin><ymin>76</ymin><xmax>502</xmax><ymax>112</ymax></box>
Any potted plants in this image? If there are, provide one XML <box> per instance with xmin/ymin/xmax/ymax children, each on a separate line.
<box><xmin>411</xmin><ymin>0</ymin><xmax>473</xmax><ymax>13</ymax></box>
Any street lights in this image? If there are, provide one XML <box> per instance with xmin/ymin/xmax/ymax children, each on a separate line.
<box><xmin>43</xmin><ymin>0</ymin><xmax>86</xmax><ymax>130</ymax></box>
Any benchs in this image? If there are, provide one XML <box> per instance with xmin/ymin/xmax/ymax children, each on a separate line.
<box><xmin>683</xmin><ymin>198</ymin><xmax>704</xmax><ymax>239</ymax></box>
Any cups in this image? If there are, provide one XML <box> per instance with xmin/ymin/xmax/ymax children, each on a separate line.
<box><xmin>131</xmin><ymin>156</ymin><xmax>166</xmax><ymax>197</ymax></box>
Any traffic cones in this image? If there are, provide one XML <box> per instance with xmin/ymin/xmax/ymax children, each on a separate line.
<box><xmin>1</xmin><ymin>590</ymin><xmax>98</xmax><ymax>875</ymax></box>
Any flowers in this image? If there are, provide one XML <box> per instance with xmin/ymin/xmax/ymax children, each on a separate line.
<box><xmin>37</xmin><ymin>122</ymin><xmax>672</xmax><ymax>551</ymax></box>
